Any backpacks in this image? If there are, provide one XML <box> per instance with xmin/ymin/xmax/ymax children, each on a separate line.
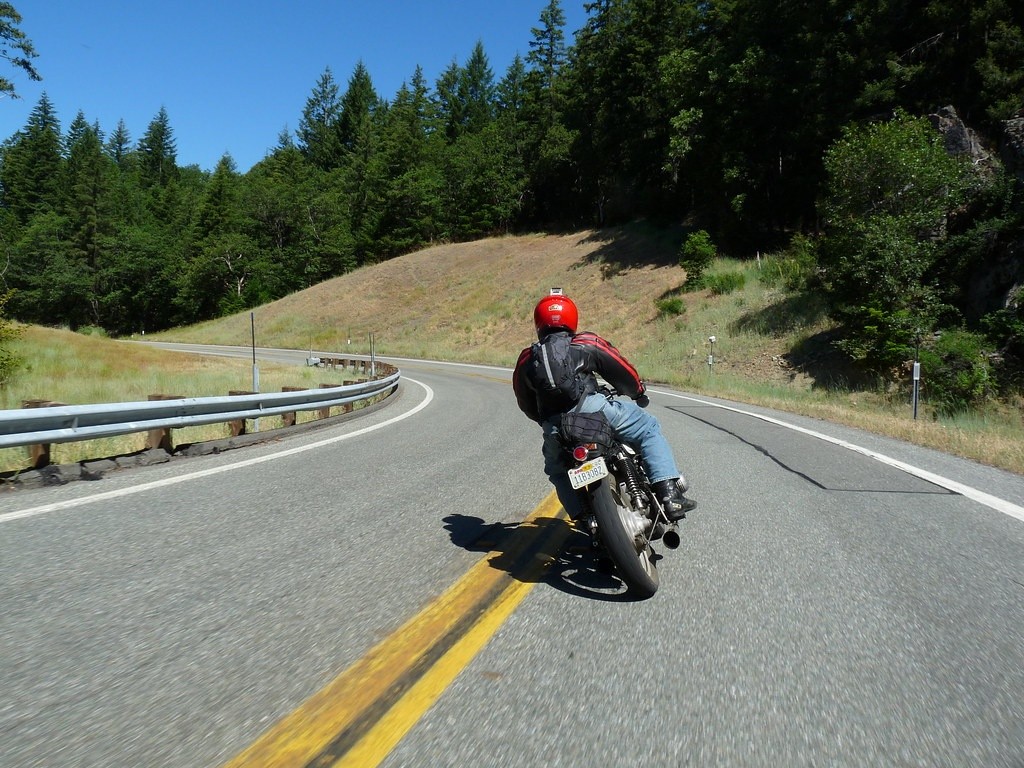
<box><xmin>531</xmin><ymin>332</ymin><xmax>590</xmax><ymax>406</ymax></box>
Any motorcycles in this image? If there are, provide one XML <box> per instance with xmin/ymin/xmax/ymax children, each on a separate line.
<box><xmin>558</xmin><ymin>378</ymin><xmax>688</xmax><ymax>598</ymax></box>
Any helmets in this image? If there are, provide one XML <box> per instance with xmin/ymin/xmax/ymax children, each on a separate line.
<box><xmin>534</xmin><ymin>295</ymin><xmax>578</xmax><ymax>333</ymax></box>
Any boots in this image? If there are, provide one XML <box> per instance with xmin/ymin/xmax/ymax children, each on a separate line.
<box><xmin>579</xmin><ymin>508</ymin><xmax>601</xmax><ymax>542</ymax></box>
<box><xmin>654</xmin><ymin>479</ymin><xmax>697</xmax><ymax>521</ymax></box>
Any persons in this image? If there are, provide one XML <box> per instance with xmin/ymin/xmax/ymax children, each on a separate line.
<box><xmin>516</xmin><ymin>296</ymin><xmax>698</xmax><ymax>530</ymax></box>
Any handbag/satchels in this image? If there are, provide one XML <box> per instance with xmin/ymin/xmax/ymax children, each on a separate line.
<box><xmin>556</xmin><ymin>411</ymin><xmax>614</xmax><ymax>448</ymax></box>
